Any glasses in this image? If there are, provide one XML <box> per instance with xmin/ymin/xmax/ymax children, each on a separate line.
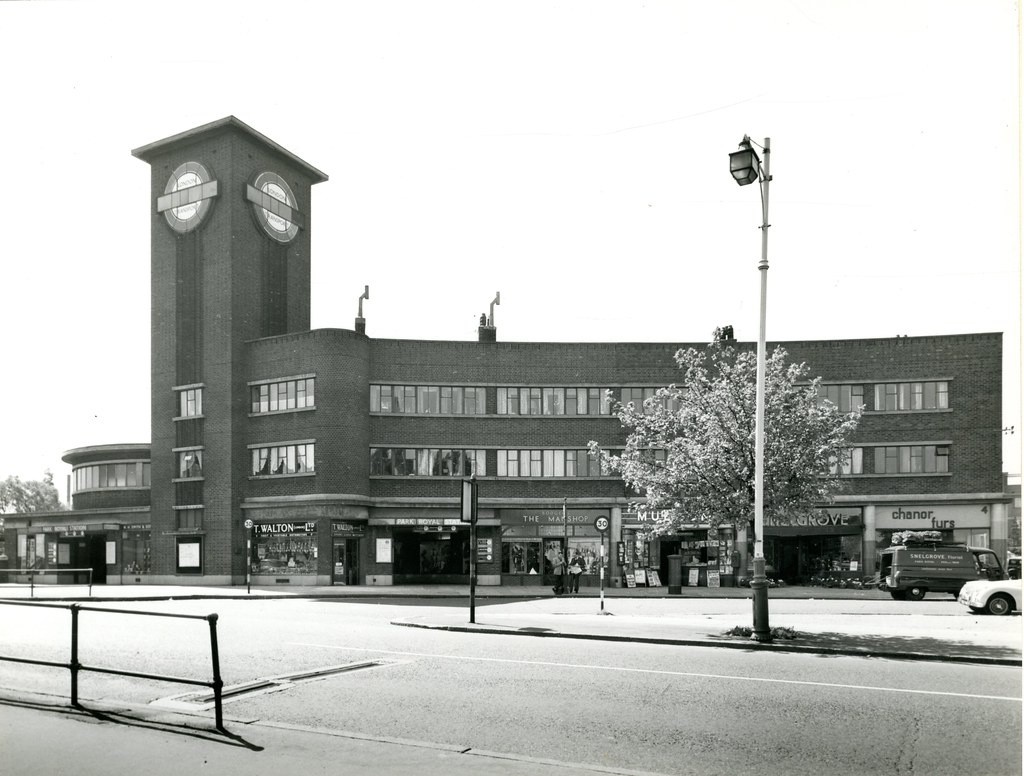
<box><xmin>558</xmin><ymin>552</ymin><xmax>563</xmax><ymax>554</ymax></box>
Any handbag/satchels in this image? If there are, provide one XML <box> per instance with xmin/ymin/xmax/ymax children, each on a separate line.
<box><xmin>569</xmin><ymin>563</ymin><xmax>582</xmax><ymax>576</ymax></box>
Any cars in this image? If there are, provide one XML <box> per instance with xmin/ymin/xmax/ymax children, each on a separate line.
<box><xmin>957</xmin><ymin>579</ymin><xmax>1022</xmax><ymax>615</ymax></box>
<box><xmin>1006</xmin><ymin>558</ymin><xmax>1022</xmax><ymax>579</ymax></box>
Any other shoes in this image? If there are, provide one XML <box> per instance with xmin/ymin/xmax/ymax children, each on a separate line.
<box><xmin>551</xmin><ymin>587</ymin><xmax>557</xmax><ymax>594</ymax></box>
<box><xmin>574</xmin><ymin>588</ymin><xmax>579</xmax><ymax>593</ymax></box>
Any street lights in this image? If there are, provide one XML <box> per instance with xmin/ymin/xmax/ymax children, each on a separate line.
<box><xmin>729</xmin><ymin>135</ymin><xmax>770</xmax><ymax>640</ymax></box>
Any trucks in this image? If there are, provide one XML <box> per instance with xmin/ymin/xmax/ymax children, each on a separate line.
<box><xmin>879</xmin><ymin>542</ymin><xmax>1004</xmax><ymax>601</ymax></box>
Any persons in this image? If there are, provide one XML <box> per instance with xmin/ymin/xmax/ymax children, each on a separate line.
<box><xmin>568</xmin><ymin>552</ymin><xmax>585</xmax><ymax>593</ymax></box>
<box><xmin>553</xmin><ymin>552</ymin><xmax>567</xmax><ymax>595</ymax></box>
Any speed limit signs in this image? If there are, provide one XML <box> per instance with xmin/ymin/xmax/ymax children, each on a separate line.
<box><xmin>594</xmin><ymin>516</ymin><xmax>610</xmax><ymax>532</ymax></box>
<box><xmin>244</xmin><ymin>519</ymin><xmax>254</xmax><ymax>529</ymax></box>
